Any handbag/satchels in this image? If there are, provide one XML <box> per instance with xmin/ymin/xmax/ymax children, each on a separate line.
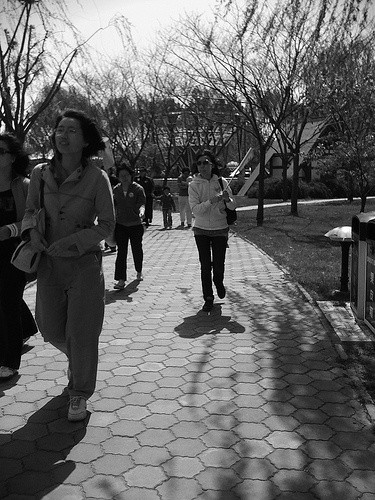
<box><xmin>224</xmin><ymin>207</ymin><xmax>237</xmax><ymax>225</ymax></box>
<box><xmin>11</xmin><ymin>207</ymin><xmax>46</xmax><ymax>273</ymax></box>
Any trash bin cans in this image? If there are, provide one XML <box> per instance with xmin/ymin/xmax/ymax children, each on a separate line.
<box><xmin>351</xmin><ymin>210</ymin><xmax>375</xmax><ymax>332</ymax></box>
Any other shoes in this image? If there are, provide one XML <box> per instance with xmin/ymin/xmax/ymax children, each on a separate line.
<box><xmin>0</xmin><ymin>366</ymin><xmax>17</xmax><ymax>377</ymax></box>
<box><xmin>163</xmin><ymin>226</ymin><xmax>172</xmax><ymax>230</ymax></box>
<box><xmin>216</xmin><ymin>282</ymin><xmax>226</xmax><ymax>299</ymax></box>
<box><xmin>137</xmin><ymin>272</ymin><xmax>143</xmax><ymax>281</ymax></box>
<box><xmin>210</xmin><ymin>262</ymin><xmax>213</xmax><ymax>267</ymax></box>
<box><xmin>142</xmin><ymin>219</ymin><xmax>152</xmax><ymax>222</ymax></box>
<box><xmin>67</xmin><ymin>395</ymin><xmax>91</xmax><ymax>422</ymax></box>
<box><xmin>181</xmin><ymin>222</ymin><xmax>185</xmax><ymax>226</ymax></box>
<box><xmin>188</xmin><ymin>224</ymin><xmax>192</xmax><ymax>227</ymax></box>
<box><xmin>22</xmin><ymin>337</ymin><xmax>30</xmax><ymax>348</ymax></box>
<box><xmin>114</xmin><ymin>283</ymin><xmax>125</xmax><ymax>289</ymax></box>
<box><xmin>203</xmin><ymin>300</ymin><xmax>213</xmax><ymax>312</ymax></box>
<box><xmin>104</xmin><ymin>247</ymin><xmax>118</xmax><ymax>252</ymax></box>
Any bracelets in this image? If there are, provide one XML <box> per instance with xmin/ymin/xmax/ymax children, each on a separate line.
<box><xmin>217</xmin><ymin>195</ymin><xmax>222</xmax><ymax>201</ymax></box>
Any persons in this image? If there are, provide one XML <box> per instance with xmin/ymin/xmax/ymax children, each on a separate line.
<box><xmin>113</xmin><ymin>163</ymin><xmax>146</xmax><ymax>289</ymax></box>
<box><xmin>187</xmin><ymin>149</ymin><xmax>237</xmax><ymax>311</ymax></box>
<box><xmin>100</xmin><ymin>162</ymin><xmax>192</xmax><ymax>252</ymax></box>
<box><xmin>21</xmin><ymin>109</ymin><xmax>116</xmax><ymax>421</ymax></box>
<box><xmin>0</xmin><ymin>134</ymin><xmax>38</xmax><ymax>379</ymax></box>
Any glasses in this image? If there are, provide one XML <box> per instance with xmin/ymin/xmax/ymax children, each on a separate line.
<box><xmin>0</xmin><ymin>147</ymin><xmax>13</xmax><ymax>154</ymax></box>
<box><xmin>55</xmin><ymin>126</ymin><xmax>82</xmax><ymax>135</ymax></box>
<box><xmin>194</xmin><ymin>160</ymin><xmax>213</xmax><ymax>166</ymax></box>
